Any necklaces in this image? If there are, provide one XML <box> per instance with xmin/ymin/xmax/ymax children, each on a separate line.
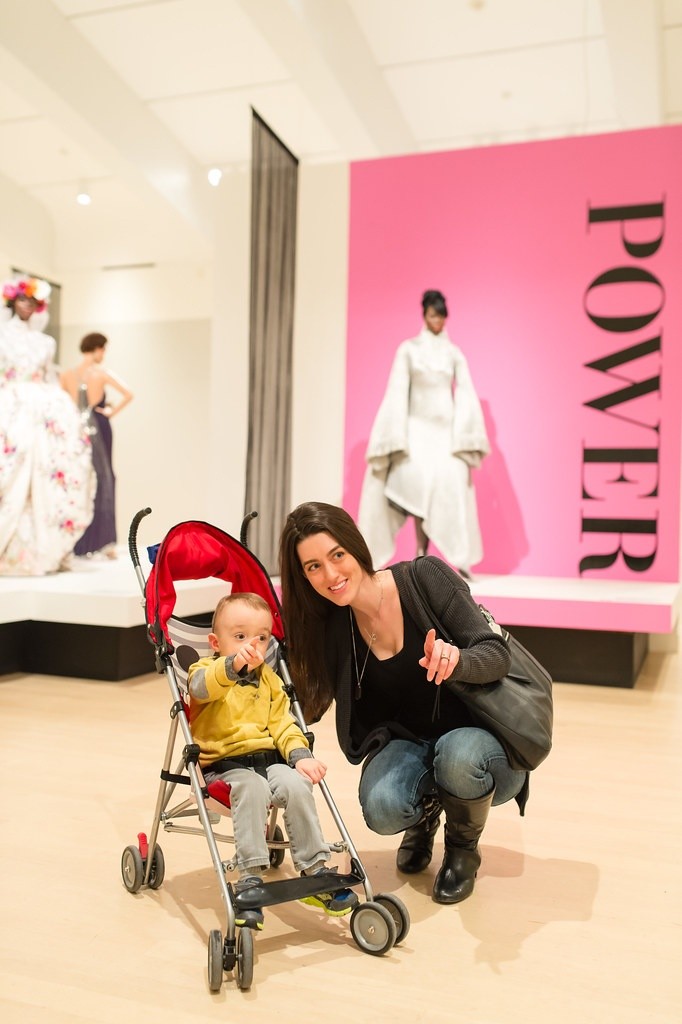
<box><xmin>349</xmin><ymin>574</ymin><xmax>383</xmax><ymax>700</ymax></box>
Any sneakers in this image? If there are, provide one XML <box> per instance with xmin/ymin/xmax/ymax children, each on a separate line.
<box><xmin>298</xmin><ymin>866</ymin><xmax>361</xmax><ymax>918</ymax></box>
<box><xmin>231</xmin><ymin>877</ymin><xmax>266</xmax><ymax>932</ymax></box>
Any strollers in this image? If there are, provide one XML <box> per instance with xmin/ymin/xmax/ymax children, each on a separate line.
<box><xmin>119</xmin><ymin>505</ymin><xmax>410</xmax><ymax>993</ymax></box>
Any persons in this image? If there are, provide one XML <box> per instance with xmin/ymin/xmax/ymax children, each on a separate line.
<box><xmin>358</xmin><ymin>289</ymin><xmax>492</xmax><ymax>585</ymax></box>
<box><xmin>58</xmin><ymin>333</ymin><xmax>133</xmax><ymax>561</ymax></box>
<box><xmin>187</xmin><ymin>592</ymin><xmax>360</xmax><ymax>931</ymax></box>
<box><xmin>0</xmin><ymin>276</ymin><xmax>99</xmax><ymax>578</ymax></box>
<box><xmin>277</xmin><ymin>502</ymin><xmax>530</xmax><ymax>905</ymax></box>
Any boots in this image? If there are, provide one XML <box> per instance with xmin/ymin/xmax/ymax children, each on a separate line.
<box><xmin>433</xmin><ymin>773</ymin><xmax>497</xmax><ymax>905</ymax></box>
<box><xmin>395</xmin><ymin>792</ymin><xmax>444</xmax><ymax>874</ymax></box>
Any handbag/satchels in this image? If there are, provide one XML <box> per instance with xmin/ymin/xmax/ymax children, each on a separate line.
<box><xmin>409</xmin><ymin>555</ymin><xmax>554</xmax><ymax>773</ymax></box>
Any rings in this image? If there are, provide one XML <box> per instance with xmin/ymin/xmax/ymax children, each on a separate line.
<box><xmin>441</xmin><ymin>653</ymin><xmax>451</xmax><ymax>661</ymax></box>
